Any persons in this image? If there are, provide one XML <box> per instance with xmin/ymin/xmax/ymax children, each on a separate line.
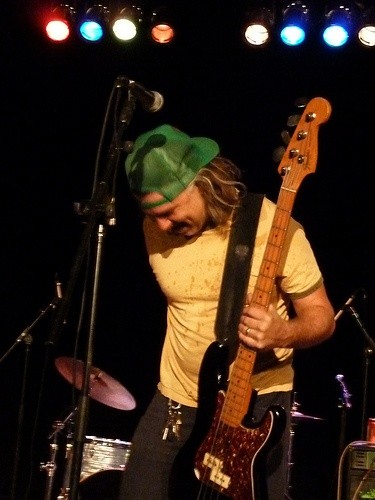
<box><xmin>120</xmin><ymin>125</ymin><xmax>335</xmax><ymax>500</ymax></box>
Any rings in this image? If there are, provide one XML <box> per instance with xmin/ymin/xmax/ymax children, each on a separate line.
<box><xmin>246</xmin><ymin>327</ymin><xmax>250</xmax><ymax>336</ymax></box>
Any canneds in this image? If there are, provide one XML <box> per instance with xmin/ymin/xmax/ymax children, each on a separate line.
<box><xmin>367</xmin><ymin>418</ymin><xmax>375</xmax><ymax>447</ymax></box>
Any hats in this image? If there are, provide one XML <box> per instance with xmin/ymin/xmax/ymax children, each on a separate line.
<box><xmin>122</xmin><ymin>123</ymin><xmax>221</xmax><ymax>210</ymax></box>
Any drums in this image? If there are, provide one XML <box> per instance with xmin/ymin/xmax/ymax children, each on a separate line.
<box><xmin>65</xmin><ymin>436</ymin><xmax>131</xmax><ymax>483</ymax></box>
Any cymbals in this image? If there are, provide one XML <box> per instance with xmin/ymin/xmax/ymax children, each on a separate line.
<box><xmin>55</xmin><ymin>356</ymin><xmax>136</xmax><ymax>411</ymax></box>
<box><xmin>291</xmin><ymin>411</ymin><xmax>324</xmax><ymax>420</ymax></box>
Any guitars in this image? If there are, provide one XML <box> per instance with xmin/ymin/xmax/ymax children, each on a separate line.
<box><xmin>193</xmin><ymin>96</ymin><xmax>331</xmax><ymax>500</ymax></box>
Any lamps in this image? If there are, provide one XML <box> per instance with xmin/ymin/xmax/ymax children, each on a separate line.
<box><xmin>44</xmin><ymin>0</ymin><xmax>375</xmax><ymax>47</ymax></box>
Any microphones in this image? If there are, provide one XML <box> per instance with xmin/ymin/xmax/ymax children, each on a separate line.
<box><xmin>334</xmin><ymin>287</ymin><xmax>363</xmax><ymax>321</ymax></box>
<box><xmin>336</xmin><ymin>374</ymin><xmax>352</xmax><ymax>407</ymax></box>
<box><xmin>54</xmin><ymin>279</ymin><xmax>64</xmax><ymax>300</ymax></box>
<box><xmin>117</xmin><ymin>76</ymin><xmax>163</xmax><ymax>113</ymax></box>
